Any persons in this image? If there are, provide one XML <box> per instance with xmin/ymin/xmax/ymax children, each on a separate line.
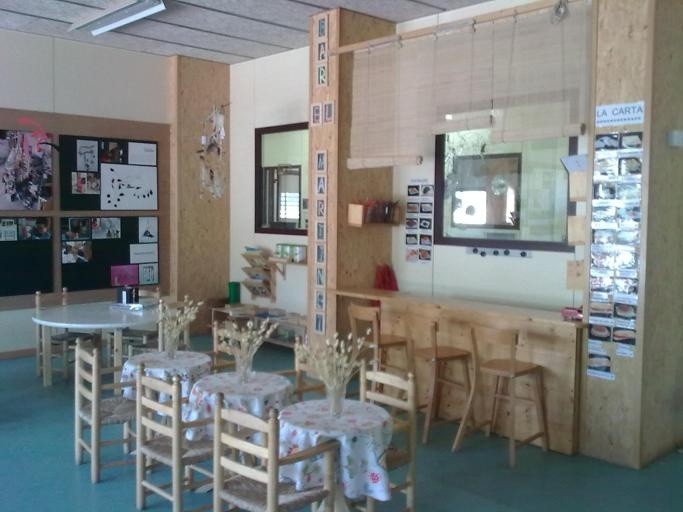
<box><xmin>22</xmin><ymin>217</ymin><xmax>51</xmax><ymax>240</ymax></box>
<box><xmin>71</xmin><ymin>241</ymin><xmax>83</xmax><ymax>257</ymax></box>
<box><xmin>61</xmin><ymin>244</ymin><xmax>76</xmax><ymax>264</ymax></box>
<box><xmin>81</xmin><ymin>242</ymin><xmax>92</xmax><ymax>263</ymax></box>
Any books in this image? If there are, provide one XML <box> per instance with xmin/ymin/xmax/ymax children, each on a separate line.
<box><xmin>110</xmin><ymin>298</ymin><xmax>158</xmax><ymax>310</ymax></box>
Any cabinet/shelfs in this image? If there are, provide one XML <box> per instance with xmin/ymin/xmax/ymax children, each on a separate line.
<box><xmin>211</xmin><ymin>304</ymin><xmax>308</xmax><ymax>349</ymax></box>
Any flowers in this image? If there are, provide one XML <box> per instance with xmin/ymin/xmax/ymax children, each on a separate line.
<box><xmin>213</xmin><ymin>310</ymin><xmax>280</xmax><ymax>380</ymax></box>
<box><xmin>151</xmin><ymin>286</ymin><xmax>204</xmax><ymax>351</ymax></box>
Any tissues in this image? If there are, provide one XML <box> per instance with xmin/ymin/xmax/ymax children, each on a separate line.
<box><xmin>116</xmin><ymin>284</ymin><xmax>140</xmax><ymax>305</ymax></box>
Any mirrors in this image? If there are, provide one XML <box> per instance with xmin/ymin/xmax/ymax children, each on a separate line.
<box><xmin>255</xmin><ymin>122</ymin><xmax>309</xmax><ymax>235</ymax></box>
<box><xmin>450</xmin><ymin>153</ymin><xmax>521</xmax><ymax>229</ymax></box>
<box><xmin>434</xmin><ymin>87</ymin><xmax>578</xmax><ymax>252</ymax></box>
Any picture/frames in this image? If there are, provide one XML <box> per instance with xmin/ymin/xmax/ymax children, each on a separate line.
<box><xmin>316</xmin><ymin>14</ymin><xmax>330</xmax><ymax>88</ymax></box>
<box><xmin>314</xmin><ymin>150</ymin><xmax>327</xmax><ymax>336</ymax></box>
<box><xmin>311</xmin><ymin>100</ymin><xmax>335</xmax><ymax>127</ymax></box>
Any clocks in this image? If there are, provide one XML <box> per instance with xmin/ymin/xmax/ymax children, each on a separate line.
<box><xmin>490</xmin><ymin>174</ymin><xmax>508</xmax><ymax>196</ymax></box>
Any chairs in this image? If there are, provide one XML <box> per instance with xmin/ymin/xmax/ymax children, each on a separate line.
<box><xmin>32</xmin><ymin>284</ymin><xmax>189</xmax><ymax>386</ymax></box>
<box><xmin>348</xmin><ymin>302</ymin><xmax>549</xmax><ymax>467</ymax></box>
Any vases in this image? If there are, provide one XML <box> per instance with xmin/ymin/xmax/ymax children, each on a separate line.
<box><xmin>164</xmin><ymin>335</ymin><xmax>178</xmax><ymax>360</ymax></box>
